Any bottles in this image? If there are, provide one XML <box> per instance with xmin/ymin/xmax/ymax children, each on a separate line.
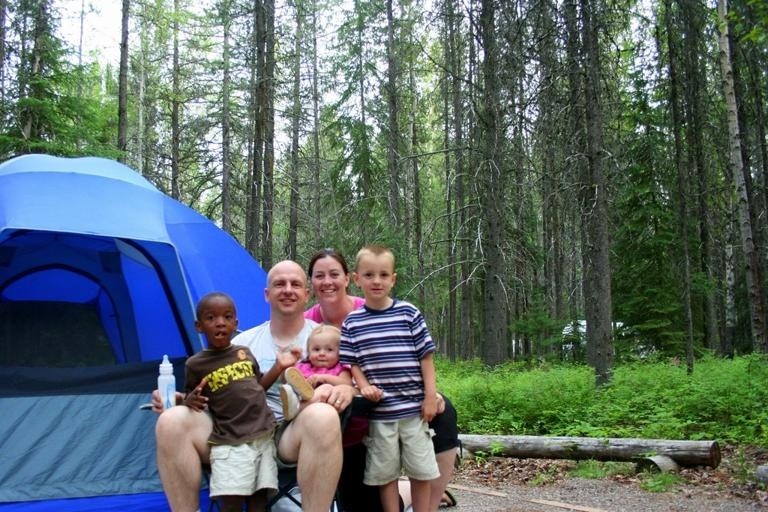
<box><xmin>157</xmin><ymin>354</ymin><xmax>176</xmax><ymax>414</ymax></box>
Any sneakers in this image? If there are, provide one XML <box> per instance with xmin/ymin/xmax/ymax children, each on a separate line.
<box><xmin>279</xmin><ymin>367</ymin><xmax>315</xmax><ymax>420</ymax></box>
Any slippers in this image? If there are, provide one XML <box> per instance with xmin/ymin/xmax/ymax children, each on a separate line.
<box><xmin>443</xmin><ymin>487</ymin><xmax>457</xmax><ymax>508</ymax></box>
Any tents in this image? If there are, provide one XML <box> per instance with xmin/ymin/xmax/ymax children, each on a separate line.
<box><xmin>0</xmin><ymin>147</ymin><xmax>339</xmax><ymax>511</ymax></box>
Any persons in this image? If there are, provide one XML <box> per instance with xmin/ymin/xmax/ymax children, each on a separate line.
<box><xmin>302</xmin><ymin>247</ymin><xmax>459</xmax><ymax>512</ymax></box>
<box><xmin>338</xmin><ymin>241</ymin><xmax>441</xmax><ymax>511</ymax></box>
<box><xmin>278</xmin><ymin>322</ymin><xmax>360</xmax><ymax>421</ymax></box>
<box><xmin>179</xmin><ymin>290</ymin><xmax>301</xmax><ymax>512</ymax></box>
<box><xmin>151</xmin><ymin>254</ymin><xmax>361</xmax><ymax>512</ymax></box>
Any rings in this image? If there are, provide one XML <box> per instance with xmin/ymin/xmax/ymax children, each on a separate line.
<box><xmin>335</xmin><ymin>399</ymin><xmax>341</xmax><ymax>405</ymax></box>
